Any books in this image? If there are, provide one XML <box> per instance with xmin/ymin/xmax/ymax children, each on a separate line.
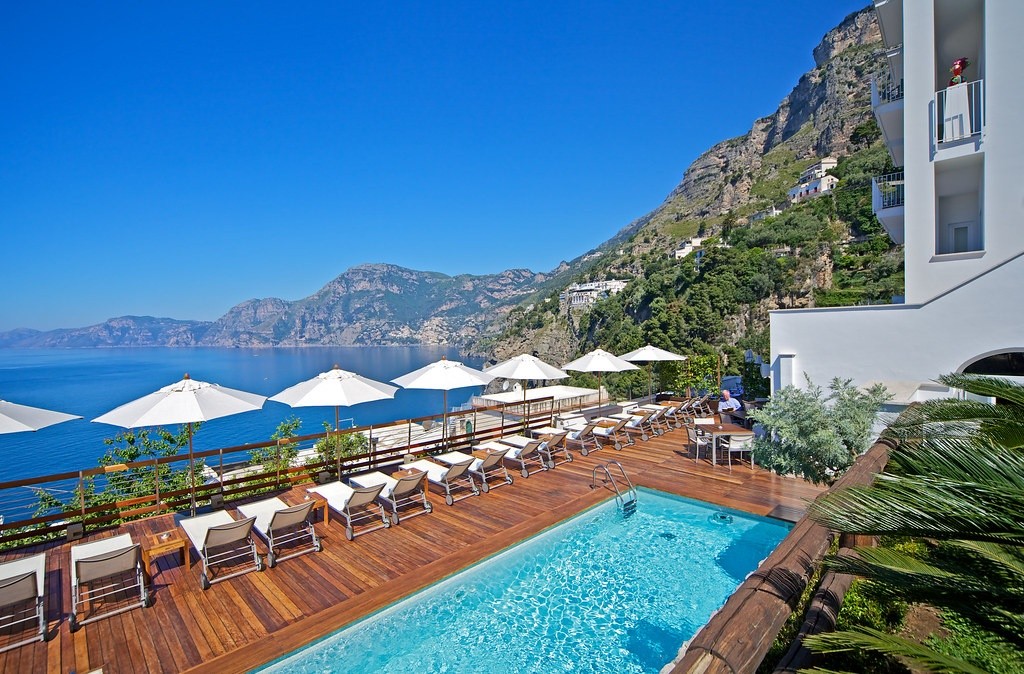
<box><xmin>722</xmin><ymin>407</ymin><xmax>734</xmax><ymax>412</ymax></box>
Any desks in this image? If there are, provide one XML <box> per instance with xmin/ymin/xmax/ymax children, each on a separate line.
<box><xmin>720</xmin><ymin>410</ymin><xmax>751</xmax><ymax>429</ymax></box>
<box><xmin>139</xmin><ymin>526</ymin><xmax>190</xmax><ymax>591</ymax></box>
<box><xmin>696</xmin><ymin>423</ymin><xmax>755</xmax><ymax>467</ymax></box>
<box><xmin>471</xmin><ymin>448</ymin><xmax>503</xmax><ymax>468</ymax></box>
<box><xmin>595</xmin><ymin>420</ymin><xmax>618</xmax><ymax>428</ymax></box>
<box><xmin>660</xmin><ymin>401</ymin><xmax>681</xmax><ymax>407</ymax></box>
<box><xmin>391</xmin><ymin>467</ymin><xmax>429</xmax><ymax>496</ymax></box>
<box><xmin>634</xmin><ymin>410</ymin><xmax>649</xmax><ymax>416</ymax></box>
<box><xmin>286</xmin><ymin>492</ymin><xmax>329</xmax><ymax>527</ymax></box>
<box><xmin>537</xmin><ymin>434</ymin><xmax>566</xmax><ymax>449</ymax></box>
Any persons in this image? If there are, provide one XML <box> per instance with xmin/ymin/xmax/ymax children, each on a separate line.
<box><xmin>717</xmin><ymin>389</ymin><xmax>743</xmax><ymax>425</ymax></box>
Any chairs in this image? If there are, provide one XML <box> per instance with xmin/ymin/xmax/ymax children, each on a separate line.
<box><xmin>1</xmin><ymin>392</ymin><xmax>714</xmax><ymax>654</ymax></box>
<box><xmin>888</xmin><ymin>78</ymin><xmax>904</xmax><ymax>103</ymax></box>
<box><xmin>718</xmin><ymin>433</ymin><xmax>754</xmax><ymax>473</ymax></box>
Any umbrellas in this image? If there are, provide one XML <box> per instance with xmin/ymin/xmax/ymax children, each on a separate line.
<box><xmin>618</xmin><ymin>342</ymin><xmax>688</xmax><ymax>404</ymax></box>
<box><xmin>0</xmin><ymin>399</ymin><xmax>85</xmax><ymax>434</ymax></box>
<box><xmin>482</xmin><ymin>354</ymin><xmax>571</xmax><ymax>437</ymax></box>
<box><xmin>267</xmin><ymin>364</ymin><xmax>400</xmax><ymax>482</ymax></box>
<box><xmin>390</xmin><ymin>355</ymin><xmax>497</xmax><ymax>453</ymax></box>
<box><xmin>560</xmin><ymin>348</ymin><xmax>641</xmax><ymax>417</ymax></box>
<box><xmin>90</xmin><ymin>373</ymin><xmax>268</xmax><ymax>517</ymax></box>
